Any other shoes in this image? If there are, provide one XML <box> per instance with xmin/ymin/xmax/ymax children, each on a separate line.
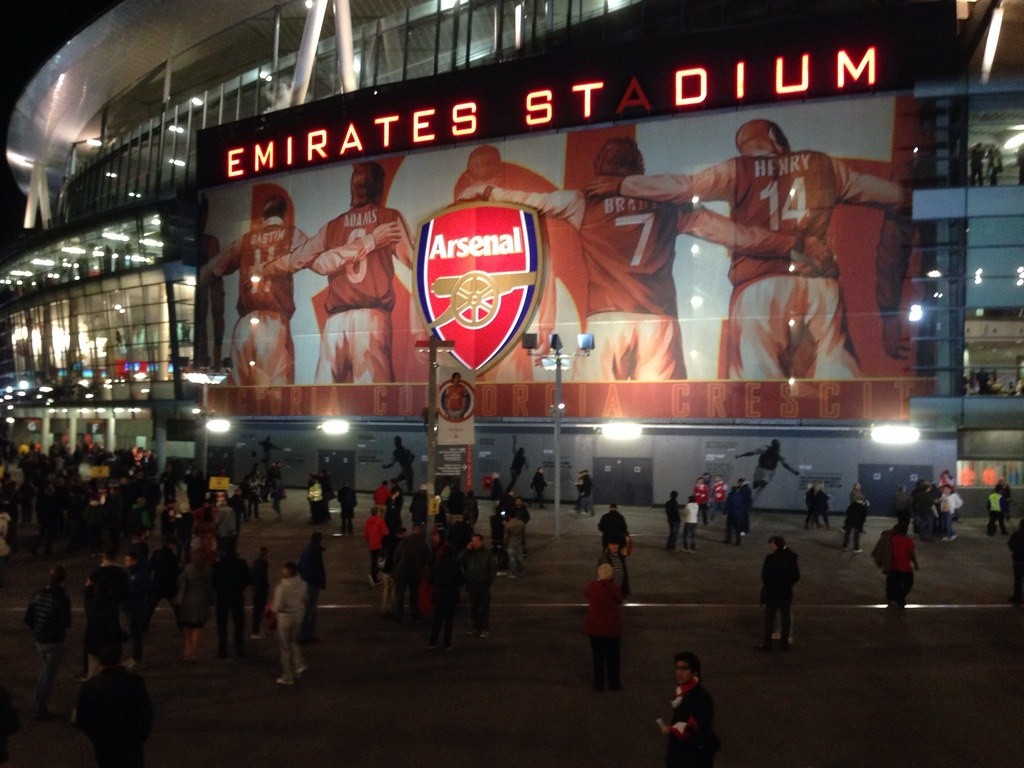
<box><xmin>687</xmin><ymin>549</ymin><xmax>695</xmax><ymax>555</ymax></box>
<box><xmin>479</xmin><ymin>631</ymin><xmax>490</xmax><ymax>637</ymax></box>
<box><xmin>943</xmin><ymin>536</ymin><xmax>951</xmax><ymax>541</ymax></box>
<box><xmin>251</xmin><ymin>633</ymin><xmax>264</xmax><ymax>639</ymax></box>
<box><xmin>444</xmin><ymin>646</ymin><xmax>453</xmax><ymax>651</ymax></box>
<box><xmin>276</xmin><ymin>678</ymin><xmax>296</xmax><ymax>685</ymax></box>
<box><xmin>463</xmin><ymin>630</ymin><xmax>472</xmax><ymax>635</ymax></box>
<box><xmin>853</xmin><ymin>549</ymin><xmax>863</xmax><ymax>554</ymax></box>
<box><xmin>423</xmin><ymin>643</ymin><xmax>436</xmax><ymax>649</ymax></box>
<box><xmin>950</xmin><ymin>532</ymin><xmax>959</xmax><ymax>540</ymax></box>
<box><xmin>496</xmin><ymin>570</ymin><xmax>508</xmax><ymax>576</ymax></box>
<box><xmin>681</xmin><ymin>547</ymin><xmax>688</xmax><ymax>553</ymax></box>
<box><xmin>293</xmin><ymin>664</ymin><xmax>309</xmax><ymax>673</ymax></box>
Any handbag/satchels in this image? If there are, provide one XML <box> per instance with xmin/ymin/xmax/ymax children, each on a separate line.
<box><xmin>620</xmin><ymin>534</ymin><xmax>632</xmax><ymax>556</ymax></box>
<box><xmin>941</xmin><ymin>501</ymin><xmax>949</xmax><ymax>512</ymax></box>
<box><xmin>930</xmin><ymin>504</ymin><xmax>939</xmax><ymax>518</ymax></box>
<box><xmin>23</xmin><ymin>599</ymin><xmax>37</xmax><ymax>627</ymax></box>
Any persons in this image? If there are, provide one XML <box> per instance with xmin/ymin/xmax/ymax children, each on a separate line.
<box><xmin>805</xmin><ymin>481</ymin><xmax>870</xmax><ymax>550</ymax></box>
<box><xmin>894</xmin><ymin>480</ymin><xmax>958</xmax><ymax>541</ymax></box>
<box><xmin>735</xmin><ymin>440</ymin><xmax>799</xmax><ymax>497</ymax></box>
<box><xmin>258</xmin><ymin>436</ymin><xmax>281</xmax><ymax>463</ymax></box>
<box><xmin>458</xmin><ymin>137</ymin><xmax>829</xmax><ymax>380</ymax></box>
<box><xmin>940</xmin><ymin>471</ymin><xmax>955</xmax><ymax>493</ymax></box>
<box><xmin>365</xmin><ymin>468</ymin><xmax>548</xmax><ymax>648</ymax></box>
<box><xmin>410</xmin><ymin>146</ymin><xmax>555</xmax><ymax>381</ymax></box>
<box><xmin>760</xmin><ymin>536</ymin><xmax>800</xmax><ymax>652</ymax></box>
<box><xmin>963</xmin><ymin>366</ymin><xmax>1024</xmax><ymax>397</ymax></box>
<box><xmin>657</xmin><ymin>653</ymin><xmax>719</xmax><ymax>768</ymax></box>
<box><xmin>383</xmin><ymin>436</ymin><xmax>415</xmax><ymax>491</ymax></box>
<box><xmin>1008</xmin><ymin>520</ymin><xmax>1024</xmax><ymax>603</ymax></box>
<box><xmin>972</xmin><ymin>142</ymin><xmax>1024</xmax><ymax>185</ymax></box>
<box><xmin>987</xmin><ymin>477</ymin><xmax>1011</xmax><ymax>535</ymax></box>
<box><xmin>587</xmin><ymin>120</ymin><xmax>906</xmax><ymax>380</ymax></box>
<box><xmin>1</xmin><ymin>437</ymin><xmax>358</xmax><ymax>768</ymax></box>
<box><xmin>665</xmin><ymin>471</ymin><xmax>752</xmax><ymax>553</ymax></box>
<box><xmin>873</xmin><ymin>522</ymin><xmax>919</xmax><ymax>608</ymax></box>
<box><xmin>570</xmin><ymin>469</ymin><xmax>594</xmax><ymax>515</ymax></box>
<box><xmin>587</xmin><ymin>504</ymin><xmax>632</xmax><ymax>689</ymax></box>
<box><xmin>506</xmin><ymin>435</ymin><xmax>528</xmax><ymax>492</ymax></box>
<box><xmin>249</xmin><ymin>162</ymin><xmax>417</xmax><ymax>384</ymax></box>
<box><xmin>201</xmin><ymin>195</ymin><xmax>401</xmax><ymax>386</ymax></box>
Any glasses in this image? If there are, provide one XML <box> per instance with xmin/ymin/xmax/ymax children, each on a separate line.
<box><xmin>673</xmin><ymin>665</ymin><xmax>691</xmax><ymax>671</ymax></box>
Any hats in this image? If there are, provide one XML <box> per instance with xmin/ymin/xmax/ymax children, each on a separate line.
<box><xmin>596</xmin><ymin>562</ymin><xmax>613</xmax><ymax>579</ymax></box>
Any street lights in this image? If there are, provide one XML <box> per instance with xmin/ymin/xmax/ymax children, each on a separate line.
<box><xmin>522</xmin><ymin>333</ymin><xmax>595</xmax><ymax>540</ymax></box>
<box><xmin>414</xmin><ymin>335</ymin><xmax>455</xmax><ymax>544</ymax></box>
<box><xmin>177</xmin><ymin>356</ymin><xmax>233</xmax><ymax>474</ymax></box>
